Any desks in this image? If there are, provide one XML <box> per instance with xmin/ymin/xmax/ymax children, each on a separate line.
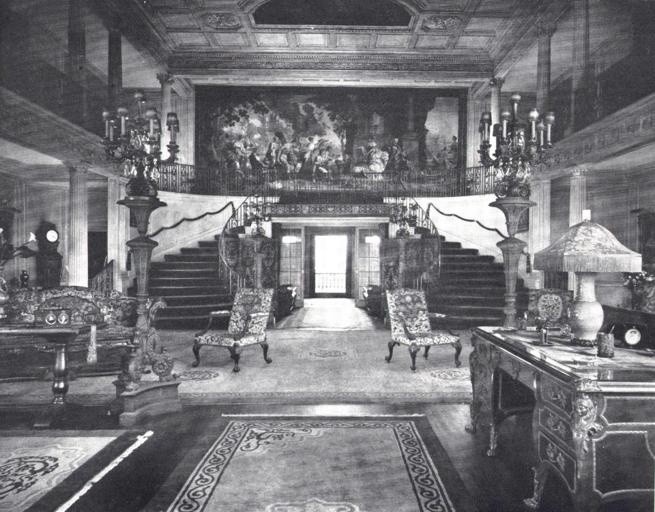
<box><xmin>465</xmin><ymin>325</ymin><xmax>655</xmax><ymax>511</ymax></box>
<box><xmin>0</xmin><ymin>321</ymin><xmax>114</xmax><ymax>429</ymax></box>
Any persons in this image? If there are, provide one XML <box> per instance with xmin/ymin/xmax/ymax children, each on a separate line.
<box><xmin>227</xmin><ymin>131</ymin><xmax>401</xmax><ymax>178</ymax></box>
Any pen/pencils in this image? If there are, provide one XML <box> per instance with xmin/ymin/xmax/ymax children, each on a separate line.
<box><xmin>609</xmin><ymin>324</ymin><xmax>616</xmax><ymax>334</ymax></box>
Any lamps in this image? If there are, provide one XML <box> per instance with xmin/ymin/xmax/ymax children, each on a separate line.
<box><xmin>533</xmin><ymin>219</ymin><xmax>643</xmax><ymax>347</ymax></box>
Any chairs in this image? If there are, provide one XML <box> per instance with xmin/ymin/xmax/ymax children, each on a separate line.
<box><xmin>384</xmin><ymin>286</ymin><xmax>463</xmax><ymax>373</ymax></box>
<box><xmin>191</xmin><ymin>285</ymin><xmax>277</xmax><ymax>373</ymax></box>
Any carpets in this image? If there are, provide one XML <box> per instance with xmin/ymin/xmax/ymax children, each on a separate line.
<box><xmin>1</xmin><ymin>428</ymin><xmax>153</xmax><ymax>512</ymax></box>
<box><xmin>139</xmin><ymin>413</ymin><xmax>482</xmax><ymax>511</ymax></box>
<box><xmin>0</xmin><ymin>327</ymin><xmax>473</xmax><ymax>407</ymax></box>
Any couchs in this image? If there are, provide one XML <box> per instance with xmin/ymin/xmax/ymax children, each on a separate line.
<box><xmin>4</xmin><ymin>286</ymin><xmax>184</xmax><ymax>426</ymax></box>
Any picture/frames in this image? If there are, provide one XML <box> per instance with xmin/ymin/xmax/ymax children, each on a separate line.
<box><xmin>193</xmin><ymin>82</ymin><xmax>469</xmax><ymax>199</ymax></box>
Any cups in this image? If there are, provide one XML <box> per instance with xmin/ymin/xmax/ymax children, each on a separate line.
<box><xmin>598</xmin><ymin>332</ymin><xmax>615</xmax><ymax>358</ymax></box>
